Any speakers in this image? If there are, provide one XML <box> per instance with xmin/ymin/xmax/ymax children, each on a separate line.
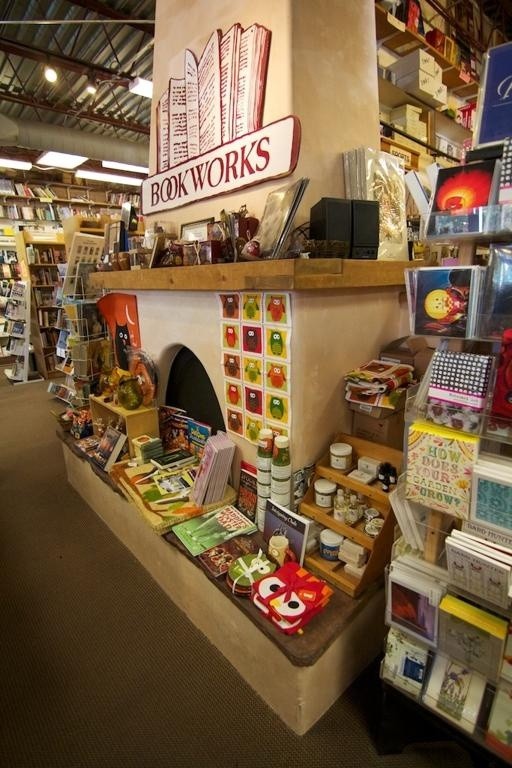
<box><xmin>350</xmin><ymin>198</ymin><xmax>381</xmax><ymax>261</ymax></box>
<box><xmin>308</xmin><ymin>196</ymin><xmax>353</xmax><ymax>262</ymax></box>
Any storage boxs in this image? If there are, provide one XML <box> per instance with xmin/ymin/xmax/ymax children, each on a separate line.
<box><xmin>349</xmin><ymin>384</ymin><xmax>417</xmax><ymax>419</ymax></box>
<box><xmin>380</xmin><ymin>335</ymin><xmax>436</xmax><ymax>379</ymax></box>
<box><xmin>396</xmin><ymin>70</ymin><xmax>433</xmax><ymax>101</ymax></box>
<box><xmin>390</xmin><ymin>105</ymin><xmax>422</xmax><ymax>136</ymax></box>
<box><xmin>379</xmin><ymin>136</ymin><xmax>419</xmax><ymax>171</ymax></box>
<box><xmin>353</xmin><ymin>410</ymin><xmax>404</xmax><ymax>449</ymax></box>
<box><xmin>427</xmin><ymin>80</ymin><xmax>448</xmax><ymax>109</ymax></box>
<box><xmin>387</xmin><ymin>50</ymin><xmax>435</xmax><ymax>74</ymax></box>
<box><xmin>434</xmin><ymin>61</ymin><xmax>444</xmax><ymax>82</ymax></box>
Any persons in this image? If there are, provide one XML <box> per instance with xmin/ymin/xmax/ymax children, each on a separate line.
<box><xmin>113</xmin><ymin>321</ymin><xmax>132</xmax><ymax>371</ymax></box>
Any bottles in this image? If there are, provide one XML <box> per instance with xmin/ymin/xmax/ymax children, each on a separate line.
<box><xmin>328</xmin><ymin>442</ymin><xmax>354</xmax><ymax>471</ymax></box>
<box><xmin>319</xmin><ymin>528</ymin><xmax>342</xmax><ymax>562</ymax></box>
<box><xmin>313</xmin><ymin>478</ymin><xmax>337</xmax><ymax>509</ymax></box>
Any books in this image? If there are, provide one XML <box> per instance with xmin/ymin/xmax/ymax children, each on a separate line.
<box><xmin>0</xmin><ymin>180</ymin><xmax>58</xmax><ymax>198</ymax></box>
<box><xmin>33</xmin><ymin>287</ymin><xmax>62</xmax><ymax>306</ymax></box>
<box><xmin>423</xmin><ymin>157</ymin><xmax>502</xmax><ymax>233</ymax></box>
<box><xmin>0</xmin><ymin>204</ymin><xmax>72</xmax><ymax>222</ymax></box>
<box><xmin>32</xmin><ymin>267</ymin><xmax>62</xmax><ymax>285</ymax></box>
<box><xmin>24</xmin><ymin>223</ymin><xmax>63</xmax><ymax>241</ymax></box>
<box><xmin>0</xmin><ymin>249</ymin><xmax>23</xmax><ymax>278</ymax></box>
<box><xmin>92</xmin><ymin>426</ymin><xmax>127</xmax><ymax>472</ymax></box>
<box><xmin>428</xmin><ymin>349</ymin><xmax>496</xmax><ymax>435</ymax></box>
<box><xmin>39</xmin><ymin>328</ymin><xmax>62</xmax><ymax>346</ymax></box>
<box><xmin>57</xmin><ymin>353</ymin><xmax>73</xmax><ymax>372</ymax></box>
<box><xmin>172</xmin><ymin>503</ymin><xmax>258</xmax><ymax>556</ymax></box>
<box><xmin>411</xmin><ymin>266</ymin><xmax>486</xmax><ymax>338</ymax></box>
<box><xmin>74</xmin><ymin>434</ymin><xmax>101</xmax><ymax>452</ymax></box>
<box><xmin>0</xmin><ymin>280</ymin><xmax>25</xmax><ymax>336</ymax></box>
<box><xmin>55</xmin><ymin>330</ymin><xmax>71</xmax><ymax>353</ymax></box>
<box><xmin>55</xmin><ymin>309</ymin><xmax>69</xmax><ymax>329</ymax></box>
<box><xmin>474</xmin><ymin>452</ymin><xmax>512</xmax><ymax>537</ymax></box>
<box><xmin>49</xmin><ymin>376</ymin><xmax>77</xmax><ymax>401</ymax></box>
<box><xmin>30</xmin><ymin>245</ymin><xmax>65</xmax><ymax>264</ymax></box>
<box><xmin>38</xmin><ymin>308</ymin><xmax>63</xmax><ymax>327</ymax></box>
<box><xmin>197</xmin><ymin>535</ymin><xmax>264</xmax><ymax>577</ymax></box>
<box><xmin>73</xmin><ymin>205</ymin><xmax>122</xmax><ymax>220</ymax></box>
<box><xmin>131</xmin><ymin>405</ymin><xmax>236</xmax><ymax>505</ymax></box>
<box><xmin>5</xmin><ymin>338</ymin><xmax>26</xmax><ymax>379</ymax></box>
<box><xmin>108</xmin><ymin>192</ymin><xmax>140</xmax><ymax>208</ymax></box>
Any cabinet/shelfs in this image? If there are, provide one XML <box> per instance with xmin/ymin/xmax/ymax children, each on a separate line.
<box><xmin>17</xmin><ymin>230</ymin><xmax>67</xmax><ymax>381</ymax></box>
<box><xmin>62</xmin><ymin>277</ymin><xmax>114</xmax><ymax>405</ymax></box>
<box><xmin>0</xmin><ymin>178</ymin><xmax>143</xmax><ymax>234</ymax></box>
<box><xmin>297</xmin><ymin>434</ymin><xmax>403</xmax><ymax>598</ymax></box>
<box><xmin>375</xmin><ymin>0</ymin><xmax>512</xmax><ymax>172</ymax></box>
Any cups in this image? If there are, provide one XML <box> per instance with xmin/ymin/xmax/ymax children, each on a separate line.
<box><xmin>269</xmin><ymin>535</ymin><xmax>296</xmax><ymax>565</ymax></box>
